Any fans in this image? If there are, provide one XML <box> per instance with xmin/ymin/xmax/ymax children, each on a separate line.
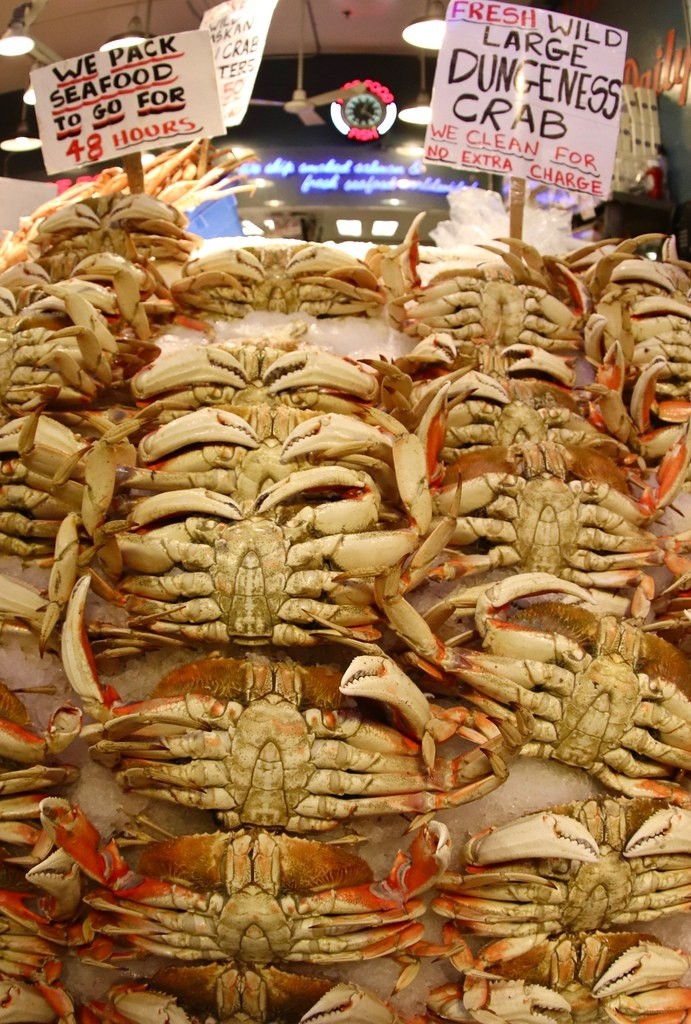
<box><xmin>250</xmin><ymin>0</ymin><xmax>365</xmax><ymax>127</ymax></box>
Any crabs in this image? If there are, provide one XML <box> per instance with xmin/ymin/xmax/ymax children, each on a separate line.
<box><xmin>355</xmin><ymin>210</ymin><xmax>691</xmax><ymax>1024</ymax></box>
<box><xmin>0</xmin><ymin>192</ymin><xmax>537</xmax><ymax>1024</ymax></box>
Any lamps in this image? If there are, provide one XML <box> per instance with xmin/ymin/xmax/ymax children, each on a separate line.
<box><xmin>0</xmin><ymin>1</ymin><xmax>40</xmax><ymax>57</ymax></box>
<box><xmin>402</xmin><ymin>0</ymin><xmax>448</xmax><ymax>50</ymax></box>
<box><xmin>397</xmin><ymin>53</ymin><xmax>438</xmax><ymax>125</ymax></box>
<box><xmin>0</xmin><ymin>106</ymin><xmax>42</xmax><ymax>152</ymax></box>
<box><xmin>23</xmin><ymin>61</ymin><xmax>47</xmax><ymax>105</ymax></box>
<box><xmin>97</xmin><ymin>0</ymin><xmax>154</xmax><ymax>52</ymax></box>
